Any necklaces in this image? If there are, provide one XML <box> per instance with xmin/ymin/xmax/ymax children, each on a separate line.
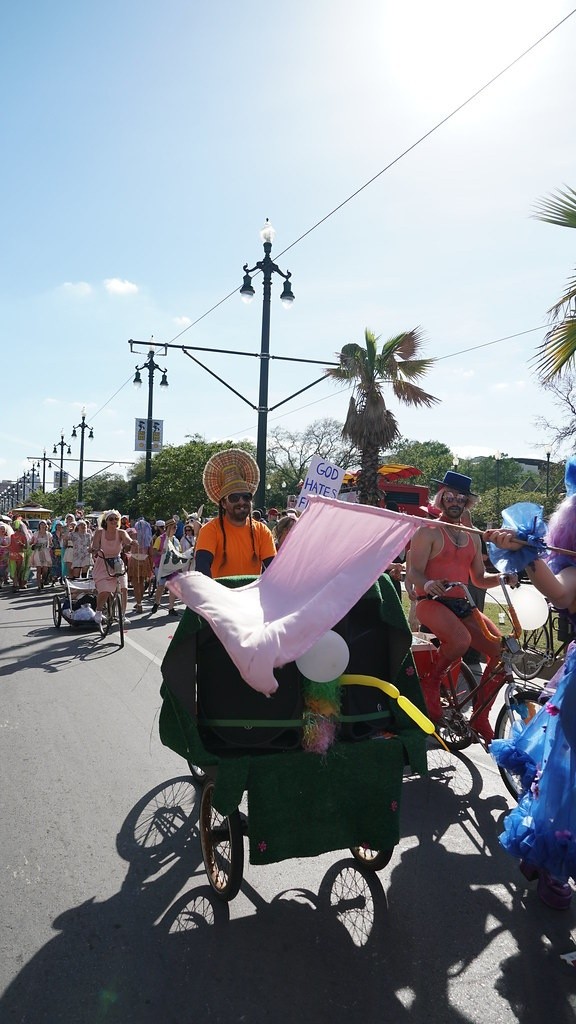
<box><xmin>446</xmin><ymin>521</ymin><xmax>463</xmax><ymax>547</ymax></box>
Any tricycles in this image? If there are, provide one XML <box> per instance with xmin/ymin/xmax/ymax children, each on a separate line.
<box><xmin>53</xmin><ymin>542</ymin><xmax>140</xmax><ymax>648</ymax></box>
<box><xmin>157</xmin><ymin>571</ymin><xmax>435</xmax><ymax>903</ymax></box>
<box><xmin>406</xmin><ymin>569</ymin><xmax>556</xmax><ymax>807</ymax></box>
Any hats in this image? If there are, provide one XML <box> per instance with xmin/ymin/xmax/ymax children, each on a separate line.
<box><xmin>164</xmin><ymin>519</ymin><xmax>176</xmax><ymax>527</ymax></box>
<box><xmin>155</xmin><ymin>520</ymin><xmax>165</xmax><ymax>526</ymax></box>
<box><xmin>430</xmin><ymin>470</ymin><xmax>479</xmax><ymax>496</ymax></box>
<box><xmin>268</xmin><ymin>508</ymin><xmax>279</xmax><ymax>515</ymax></box>
<box><xmin>202</xmin><ymin>448</ymin><xmax>260</xmax><ymax>504</ymax></box>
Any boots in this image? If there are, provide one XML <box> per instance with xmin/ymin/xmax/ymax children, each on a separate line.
<box><xmin>419</xmin><ymin>646</ymin><xmax>455</xmax><ymax>722</ymax></box>
<box><xmin>468</xmin><ymin>668</ymin><xmax>506</xmax><ymax>743</ymax></box>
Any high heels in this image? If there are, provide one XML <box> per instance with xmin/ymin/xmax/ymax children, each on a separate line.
<box><xmin>518</xmin><ymin>859</ymin><xmax>571</xmax><ymax>910</ymax></box>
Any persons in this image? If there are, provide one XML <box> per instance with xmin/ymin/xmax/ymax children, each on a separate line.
<box><xmin>405</xmin><ymin>472</ymin><xmax>518</xmax><ymax>748</ymax></box>
<box><xmin>0</xmin><ymin>508</ymin><xmax>219</xmax><ymax>625</ymax></box>
<box><xmin>249</xmin><ymin>494</ymin><xmax>303</xmax><ymax>552</ymax></box>
<box><xmin>385</xmin><ymin>557</ymin><xmax>403</xmax><ymax>605</ymax></box>
<box><xmin>482</xmin><ymin>456</ymin><xmax>576</xmax><ymax>909</ymax></box>
<box><xmin>385</xmin><ymin>501</ymin><xmax>398</xmax><ymax>511</ymax></box>
<box><xmin>194</xmin><ymin>448</ymin><xmax>278</xmax><ymax>579</ymax></box>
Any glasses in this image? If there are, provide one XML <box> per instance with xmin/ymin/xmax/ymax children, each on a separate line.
<box><xmin>108</xmin><ymin>517</ymin><xmax>119</xmax><ymax>521</ymax></box>
<box><xmin>443</xmin><ymin>490</ymin><xmax>465</xmax><ymax>503</ymax></box>
<box><xmin>223</xmin><ymin>492</ymin><xmax>253</xmax><ymax>503</ymax></box>
<box><xmin>185</xmin><ymin>529</ymin><xmax>192</xmax><ymax>532</ymax></box>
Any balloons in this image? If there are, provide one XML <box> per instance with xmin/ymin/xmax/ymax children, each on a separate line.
<box><xmin>340</xmin><ymin>674</ymin><xmax>452</xmax><ymax>754</ymax></box>
<box><xmin>292</xmin><ymin>628</ymin><xmax>350</xmax><ymax>683</ymax></box>
<box><xmin>512</xmin><ymin>584</ymin><xmax>549</xmax><ymax>631</ymax></box>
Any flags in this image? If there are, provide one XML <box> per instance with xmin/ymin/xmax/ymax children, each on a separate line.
<box><xmin>165</xmin><ymin>499</ymin><xmax>422</xmax><ymax>693</ymax></box>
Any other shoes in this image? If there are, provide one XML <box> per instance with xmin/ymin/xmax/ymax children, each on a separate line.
<box><xmin>151</xmin><ymin>603</ymin><xmax>160</xmax><ymax>613</ymax></box>
<box><xmin>95</xmin><ymin>610</ymin><xmax>102</xmax><ymax>623</ymax></box>
<box><xmin>134</xmin><ymin>604</ymin><xmax>142</xmax><ymax>613</ymax></box>
<box><xmin>121</xmin><ymin>617</ymin><xmax>130</xmax><ymax>624</ymax></box>
<box><xmin>169</xmin><ymin>609</ymin><xmax>178</xmax><ymax>616</ymax></box>
<box><xmin>0</xmin><ymin>574</ymin><xmax>65</xmax><ymax>593</ymax></box>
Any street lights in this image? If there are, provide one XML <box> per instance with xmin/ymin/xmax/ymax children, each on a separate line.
<box><xmin>0</xmin><ymin>448</ymin><xmax>52</xmax><ymax>516</ymax></box>
<box><xmin>133</xmin><ymin>334</ymin><xmax>170</xmax><ymax>520</ymax></box>
<box><xmin>281</xmin><ymin>480</ymin><xmax>286</xmax><ymax>509</ymax></box>
<box><xmin>239</xmin><ymin>218</ymin><xmax>296</xmax><ymax>517</ymax></box>
<box><xmin>69</xmin><ymin>404</ymin><xmax>94</xmax><ymax>506</ymax></box>
<box><xmin>494</xmin><ymin>448</ymin><xmax>502</xmax><ymax>524</ymax></box>
<box><xmin>52</xmin><ymin>427</ymin><xmax>72</xmax><ymax>513</ymax></box>
<box><xmin>545</xmin><ymin>444</ymin><xmax>554</xmax><ymax>497</ymax></box>
<box><xmin>266</xmin><ymin>483</ymin><xmax>272</xmax><ymax>509</ymax></box>
